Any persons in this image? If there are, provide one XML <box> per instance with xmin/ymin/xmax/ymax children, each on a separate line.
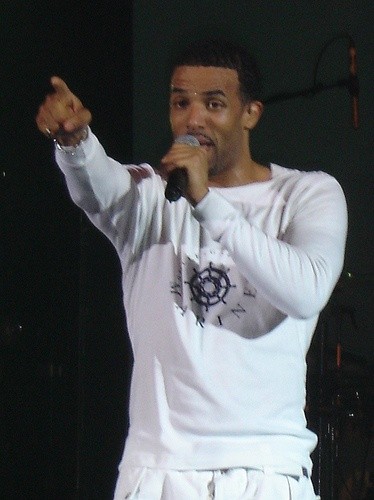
<box><xmin>35</xmin><ymin>39</ymin><xmax>349</xmax><ymax>500</ymax></box>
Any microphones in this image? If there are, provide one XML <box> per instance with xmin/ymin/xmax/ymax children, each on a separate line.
<box><xmin>164</xmin><ymin>134</ymin><xmax>200</xmax><ymax>203</ymax></box>
<box><xmin>348</xmin><ymin>41</ymin><xmax>361</xmax><ymax>131</ymax></box>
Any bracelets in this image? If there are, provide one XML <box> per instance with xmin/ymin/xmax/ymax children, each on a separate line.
<box><xmin>52</xmin><ymin>129</ymin><xmax>87</xmax><ymax>157</ymax></box>
